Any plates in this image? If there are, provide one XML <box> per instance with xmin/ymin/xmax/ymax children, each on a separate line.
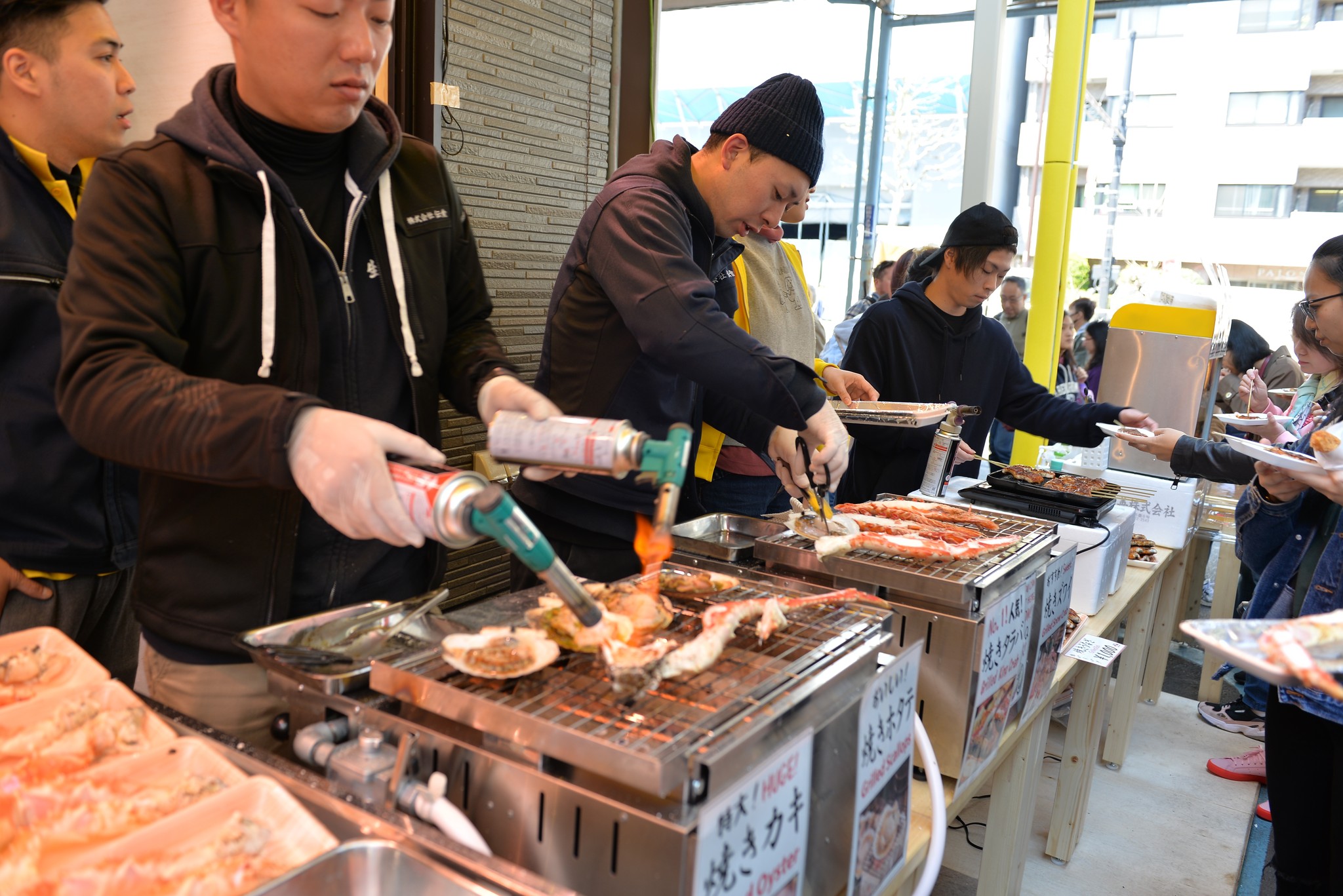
<box><xmin>1096</xmin><ymin>422</ymin><xmax>1155</xmax><ymax>443</ymax></box>
<box><xmin>1316</xmin><ymin>415</ymin><xmax>1327</xmax><ymax>421</ymax></box>
<box><xmin>1212</xmin><ymin>413</ymin><xmax>1296</xmax><ymax>426</ymax></box>
<box><xmin>1267</xmin><ymin>388</ymin><xmax>1298</xmax><ymax>396</ymax></box>
<box><xmin>1060</xmin><ymin>612</ymin><xmax>1088</xmax><ymax>653</ymax></box>
<box><xmin>1178</xmin><ymin>617</ymin><xmax>1305</xmax><ymax>686</ymax></box>
<box><xmin>1127</xmin><ymin>555</ymin><xmax>1160</xmax><ymax>569</ymax></box>
<box><xmin>1224</xmin><ymin>433</ymin><xmax>1330</xmax><ymax>477</ymax></box>
<box><xmin>0</xmin><ymin>627</ymin><xmax>341</xmax><ymax>896</ymax></box>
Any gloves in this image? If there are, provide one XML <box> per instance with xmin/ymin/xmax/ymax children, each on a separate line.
<box><xmin>289</xmin><ymin>405</ymin><xmax>447</xmax><ymax>547</ymax></box>
<box><xmin>799</xmin><ymin>398</ymin><xmax>851</xmax><ymax>494</ymax></box>
<box><xmin>767</xmin><ymin>425</ymin><xmax>810</xmax><ymax>512</ymax></box>
<box><xmin>477</xmin><ymin>375</ymin><xmax>577</xmax><ymax>481</ymax></box>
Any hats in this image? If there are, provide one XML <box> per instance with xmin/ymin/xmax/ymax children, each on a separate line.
<box><xmin>709</xmin><ymin>73</ymin><xmax>825</xmax><ymax>189</ymax></box>
<box><xmin>919</xmin><ymin>201</ymin><xmax>1018</xmax><ymax>269</ymax></box>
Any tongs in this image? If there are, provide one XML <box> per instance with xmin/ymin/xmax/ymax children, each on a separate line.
<box><xmin>300</xmin><ymin>587</ymin><xmax>450</xmax><ymax>662</ymax></box>
<box><xmin>775</xmin><ymin>457</ymin><xmax>834</xmax><ymax>521</ymax></box>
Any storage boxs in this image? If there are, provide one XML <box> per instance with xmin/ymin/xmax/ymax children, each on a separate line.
<box><xmin>1062</xmin><ymin>463</ymin><xmax>1198</xmax><ymax>549</ymax></box>
<box><xmin>907</xmin><ymin>476</ymin><xmax>1135</xmax><ymax>616</ymax></box>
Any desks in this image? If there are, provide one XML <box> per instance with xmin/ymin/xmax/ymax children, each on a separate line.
<box><xmin>883</xmin><ymin>529</ymin><xmax>1241</xmax><ymax>896</ymax></box>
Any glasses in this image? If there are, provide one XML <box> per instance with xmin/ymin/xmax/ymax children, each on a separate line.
<box><xmin>1298</xmin><ymin>292</ymin><xmax>1343</xmax><ymax>321</ymax></box>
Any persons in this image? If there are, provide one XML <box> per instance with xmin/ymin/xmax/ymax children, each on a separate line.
<box><xmin>56</xmin><ymin>0</ymin><xmax>577</xmax><ymax>755</ymax></box>
<box><xmin>832</xmin><ymin>202</ymin><xmax>1159</xmax><ymax>514</ymax></box>
<box><xmin>1233</xmin><ymin>233</ymin><xmax>1343</xmax><ymax>895</ymax></box>
<box><xmin>509</xmin><ymin>71</ymin><xmax>851</xmax><ymax>591</ymax></box>
<box><xmin>1116</xmin><ymin>372</ymin><xmax>1311</xmax><ymax>819</ymax></box>
<box><xmin>1197</xmin><ymin>298</ymin><xmax>1343</xmax><ymax>743</ymax></box>
<box><xmin>0</xmin><ymin>0</ymin><xmax>140</xmax><ymax>693</ymax></box>
<box><xmin>695</xmin><ymin>180</ymin><xmax>878</xmax><ymax>519</ymax></box>
<box><xmin>1200</xmin><ymin>404</ymin><xmax>1228</xmax><ymax>608</ymax></box>
<box><xmin>987</xmin><ymin>275</ymin><xmax>1027</xmax><ymax>473</ymax></box>
<box><xmin>818</xmin><ymin>245</ymin><xmax>939</xmax><ymax>506</ymax></box>
<box><xmin>1049</xmin><ymin>298</ymin><xmax>1109</xmax><ymax>446</ymax></box>
<box><xmin>1218</xmin><ymin>315</ymin><xmax>1306</xmax><ymax>620</ymax></box>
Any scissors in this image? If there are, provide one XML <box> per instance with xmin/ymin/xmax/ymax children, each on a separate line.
<box><xmin>794</xmin><ymin>436</ymin><xmax>833</xmax><ymax>536</ymax></box>
<box><xmin>261</xmin><ymin>641</ymin><xmax>407</xmax><ymax>671</ymax></box>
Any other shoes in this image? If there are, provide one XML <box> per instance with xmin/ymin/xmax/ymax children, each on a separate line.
<box><xmin>1233</xmin><ymin>670</ymin><xmax>1246</xmax><ymax>684</ymax></box>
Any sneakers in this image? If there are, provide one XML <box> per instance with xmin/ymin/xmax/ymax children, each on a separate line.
<box><xmin>1257</xmin><ymin>800</ymin><xmax>1273</xmax><ymax>822</ymax></box>
<box><xmin>1197</xmin><ymin>694</ymin><xmax>1266</xmax><ymax>741</ymax></box>
<box><xmin>1200</xmin><ymin>578</ymin><xmax>1214</xmax><ymax>605</ymax></box>
<box><xmin>1205</xmin><ymin>744</ymin><xmax>1268</xmax><ymax>785</ymax></box>
